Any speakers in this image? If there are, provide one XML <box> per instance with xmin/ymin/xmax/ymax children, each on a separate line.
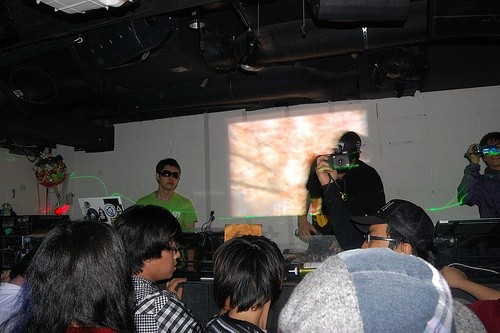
<box><xmin>308</xmin><ymin>0</ymin><xmax>411</xmax><ymax>22</ymax></box>
<box><xmin>426</xmin><ymin>0</ymin><xmax>500</xmax><ymax>41</ymax></box>
<box><xmin>0</xmin><ymin>32</ymin><xmax>106</xmax><ymax>119</ymax></box>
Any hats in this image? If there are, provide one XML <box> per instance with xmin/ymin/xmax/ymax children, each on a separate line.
<box><xmin>349</xmin><ymin>199</ymin><xmax>434</xmax><ymax>249</ymax></box>
<box><xmin>277</xmin><ymin>247</ymin><xmax>488</xmax><ymax>333</ymax></box>
<box><xmin>336</xmin><ymin>131</ymin><xmax>361</xmax><ymax>155</ymax></box>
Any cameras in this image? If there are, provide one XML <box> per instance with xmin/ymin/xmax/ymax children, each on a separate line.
<box><xmin>324</xmin><ymin>144</ymin><xmax>349</xmax><ymax>169</ymax></box>
<box><xmin>473</xmin><ymin>145</ymin><xmax>498</xmax><ymax>156</ymax></box>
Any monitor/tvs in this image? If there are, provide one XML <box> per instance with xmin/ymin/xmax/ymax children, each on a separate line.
<box><xmin>78</xmin><ymin>196</ymin><xmax>124</xmax><ymax>226</ymax></box>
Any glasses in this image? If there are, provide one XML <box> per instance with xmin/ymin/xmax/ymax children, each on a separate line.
<box><xmin>362</xmin><ymin>234</ymin><xmax>398</xmax><ymax>243</ymax></box>
<box><xmin>157</xmin><ymin>170</ymin><xmax>180</xmax><ymax>178</ymax></box>
<box><xmin>168</xmin><ymin>244</ymin><xmax>181</xmax><ymax>251</ymax></box>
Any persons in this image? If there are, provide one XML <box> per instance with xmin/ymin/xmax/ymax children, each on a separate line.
<box><xmin>111</xmin><ymin>205</ymin><xmax>205</xmax><ymax>333</ymax></box>
<box><xmin>351</xmin><ymin>198</ymin><xmax>500</xmax><ymax>333</ymax></box>
<box><xmin>201</xmin><ymin>234</ymin><xmax>286</xmax><ymax>333</ymax></box>
<box><xmin>456</xmin><ymin>132</ymin><xmax>500</xmax><ymax>218</ymax></box>
<box><xmin>134</xmin><ymin>158</ymin><xmax>199</xmax><ymax>270</ymax></box>
<box><xmin>297</xmin><ymin>131</ymin><xmax>386</xmax><ymax>262</ymax></box>
<box><xmin>0</xmin><ymin>219</ymin><xmax>137</xmax><ymax>333</ymax></box>
<box><xmin>277</xmin><ymin>246</ymin><xmax>488</xmax><ymax>333</ymax></box>
<box><xmin>0</xmin><ymin>245</ymin><xmax>41</xmax><ymax>333</ymax></box>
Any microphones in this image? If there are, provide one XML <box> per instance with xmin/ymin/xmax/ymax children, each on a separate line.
<box><xmin>204</xmin><ymin>216</ymin><xmax>215</xmax><ymax>232</ymax></box>
<box><xmin>202</xmin><ymin>211</ymin><xmax>214</xmax><ymax>232</ymax></box>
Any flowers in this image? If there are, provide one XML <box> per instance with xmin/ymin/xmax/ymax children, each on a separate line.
<box><xmin>32</xmin><ymin>155</ymin><xmax>68</xmax><ymax>203</ymax></box>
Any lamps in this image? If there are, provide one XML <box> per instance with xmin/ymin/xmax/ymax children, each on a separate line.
<box><xmin>189</xmin><ymin>11</ymin><xmax>205</xmax><ymax>29</ymax></box>
<box><xmin>240</xmin><ymin>22</ymin><xmax>264</xmax><ymax>73</ymax></box>
